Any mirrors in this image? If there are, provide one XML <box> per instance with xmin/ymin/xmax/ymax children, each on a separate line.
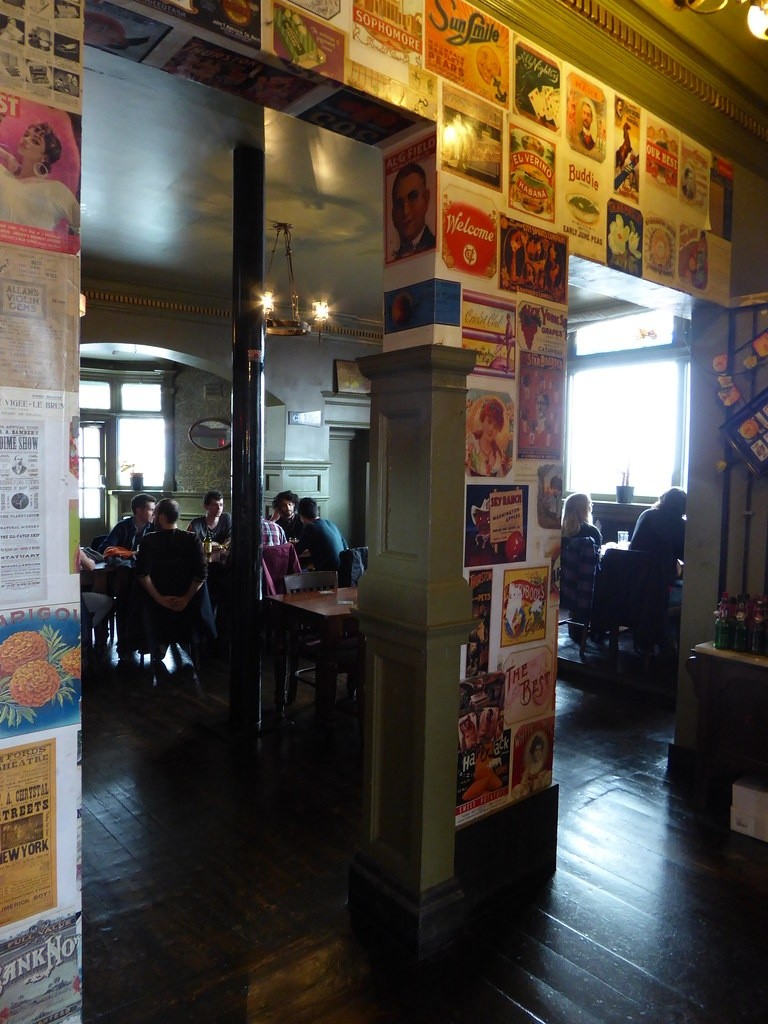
<box><xmin>188</xmin><ymin>418</ymin><xmax>231</xmax><ymax>452</ymax></box>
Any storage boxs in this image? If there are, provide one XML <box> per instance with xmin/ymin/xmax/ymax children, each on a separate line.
<box><xmin>730</xmin><ymin>806</ymin><xmax>768</xmax><ymax>842</ymax></box>
<box><xmin>732</xmin><ymin>776</ymin><xmax>768</xmax><ymax>818</ymax></box>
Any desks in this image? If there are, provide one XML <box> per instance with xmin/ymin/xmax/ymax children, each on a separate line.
<box><xmin>264</xmin><ymin>586</ymin><xmax>366</xmax><ymax>728</ymax></box>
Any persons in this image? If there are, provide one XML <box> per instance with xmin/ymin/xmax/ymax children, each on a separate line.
<box><xmin>80</xmin><ymin>490</ymin><xmax>347</xmax><ymax>668</ymax></box>
<box><xmin>563</xmin><ymin>490</ymin><xmax>685</xmax><ymax>648</ymax></box>
<box><xmin>0</xmin><ymin>120</ymin><xmax>80</xmax><ymax>233</ymax></box>
<box><xmin>388</xmin><ymin>165</ymin><xmax>435</xmax><ymax>261</ymax></box>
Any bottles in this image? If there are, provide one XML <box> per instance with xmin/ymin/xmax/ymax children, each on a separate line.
<box><xmin>710</xmin><ymin>594</ymin><xmax>768</xmax><ymax>654</ymax></box>
<box><xmin>204</xmin><ymin>531</ymin><xmax>212</xmax><ymax>554</ymax></box>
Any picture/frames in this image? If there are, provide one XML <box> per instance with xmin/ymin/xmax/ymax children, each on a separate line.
<box><xmin>718</xmin><ymin>387</ymin><xmax>768</xmax><ymax>480</ymax></box>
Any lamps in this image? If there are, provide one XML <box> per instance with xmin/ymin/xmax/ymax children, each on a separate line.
<box><xmin>265</xmin><ymin>222</ymin><xmax>330</xmax><ymax>336</ymax></box>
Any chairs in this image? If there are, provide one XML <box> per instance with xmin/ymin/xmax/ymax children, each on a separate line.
<box><xmin>558</xmin><ymin>536</ymin><xmax>600</xmax><ymax>657</ymax></box>
<box><xmin>282</xmin><ymin>571</ymin><xmax>356</xmax><ymax>703</ymax></box>
<box><xmin>590</xmin><ymin>548</ymin><xmax>681</xmax><ymax>675</ymax></box>
<box><xmin>339</xmin><ymin>546</ymin><xmax>368</xmax><ymax>586</ymax></box>
<box><xmin>124</xmin><ymin>573</ymin><xmax>218</xmax><ymax>687</ymax></box>
<box><xmin>263</xmin><ymin>543</ymin><xmax>302</xmax><ymax>595</ymax></box>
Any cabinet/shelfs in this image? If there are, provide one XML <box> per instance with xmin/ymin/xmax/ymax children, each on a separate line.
<box><xmin>685</xmin><ymin>640</ymin><xmax>768</xmax><ymax>815</ymax></box>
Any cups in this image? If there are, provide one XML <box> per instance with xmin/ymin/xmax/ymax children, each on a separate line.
<box><xmin>617</xmin><ymin>531</ymin><xmax>628</xmax><ymax>549</ymax></box>
<box><xmin>289</xmin><ymin>537</ymin><xmax>296</xmax><ymax>542</ymax></box>
